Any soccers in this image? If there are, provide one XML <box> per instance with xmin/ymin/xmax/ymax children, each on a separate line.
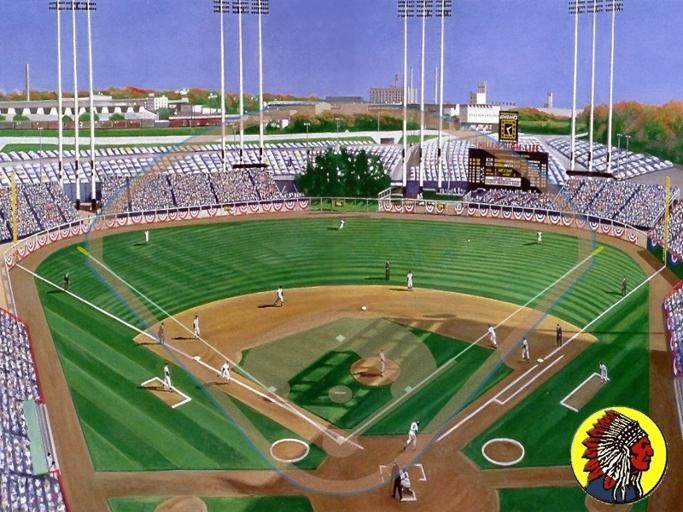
<box><xmin>362</xmin><ymin>306</ymin><xmax>366</xmax><ymax>311</ymax></box>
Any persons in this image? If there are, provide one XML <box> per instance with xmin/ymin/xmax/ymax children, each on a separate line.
<box><xmin>376</xmin><ymin>349</ymin><xmax>387</xmax><ymax>376</ymax></box>
<box><xmin>162</xmin><ymin>363</ymin><xmax>172</xmax><ymax>392</ymax></box>
<box><xmin>215</xmin><ymin>360</ymin><xmax>230</xmax><ymax>382</ymax></box>
<box><xmin>580</xmin><ymin>409</ymin><xmax>653</xmax><ymax>503</ymax></box>
<box><xmin>1</xmin><ymin>166</ymin><xmax>305</xmax><ymax>243</ymax></box>
<box><xmin>401</xmin><ymin>468</ymin><xmax>413</xmax><ymax>496</ymax></box>
<box><xmin>401</xmin><ymin>420</ymin><xmax>420</xmax><ymax>453</ymax></box>
<box><xmin>390</xmin><ymin>461</ymin><xmax>402</xmax><ymax>500</ymax></box>
<box><xmin>519</xmin><ymin>336</ymin><xmax>530</xmax><ymax>362</ymax></box>
<box><xmin>472</xmin><ymin>177</ymin><xmax>682</xmax><ymax>256</ymax></box>
<box><xmin>63</xmin><ymin>270</ymin><xmax>70</xmax><ymax>290</ymax></box>
<box><xmin>158</xmin><ymin>322</ymin><xmax>166</xmax><ymax>345</ymax></box>
<box><xmin>405</xmin><ymin>270</ymin><xmax>414</xmax><ymax>290</ymax></box>
<box><xmin>273</xmin><ymin>285</ymin><xmax>284</xmax><ymax>308</ymax></box>
<box><xmin>192</xmin><ymin>314</ymin><xmax>200</xmax><ymax>338</ymax></box>
<box><xmin>385</xmin><ymin>258</ymin><xmax>390</xmax><ymax>280</ymax></box>
<box><xmin>0</xmin><ymin>308</ymin><xmax>70</xmax><ymax>511</ymax></box>
<box><xmin>487</xmin><ymin>324</ymin><xmax>498</xmax><ymax>350</ymax></box>
<box><xmin>597</xmin><ymin>360</ymin><xmax>607</xmax><ymax>385</ymax></box>
<box><xmin>555</xmin><ymin>324</ymin><xmax>562</xmax><ymax>347</ymax></box>
<box><xmin>661</xmin><ymin>284</ymin><xmax>682</xmax><ymax>379</ymax></box>
<box><xmin>620</xmin><ymin>275</ymin><xmax>626</xmax><ymax>296</ymax></box>
